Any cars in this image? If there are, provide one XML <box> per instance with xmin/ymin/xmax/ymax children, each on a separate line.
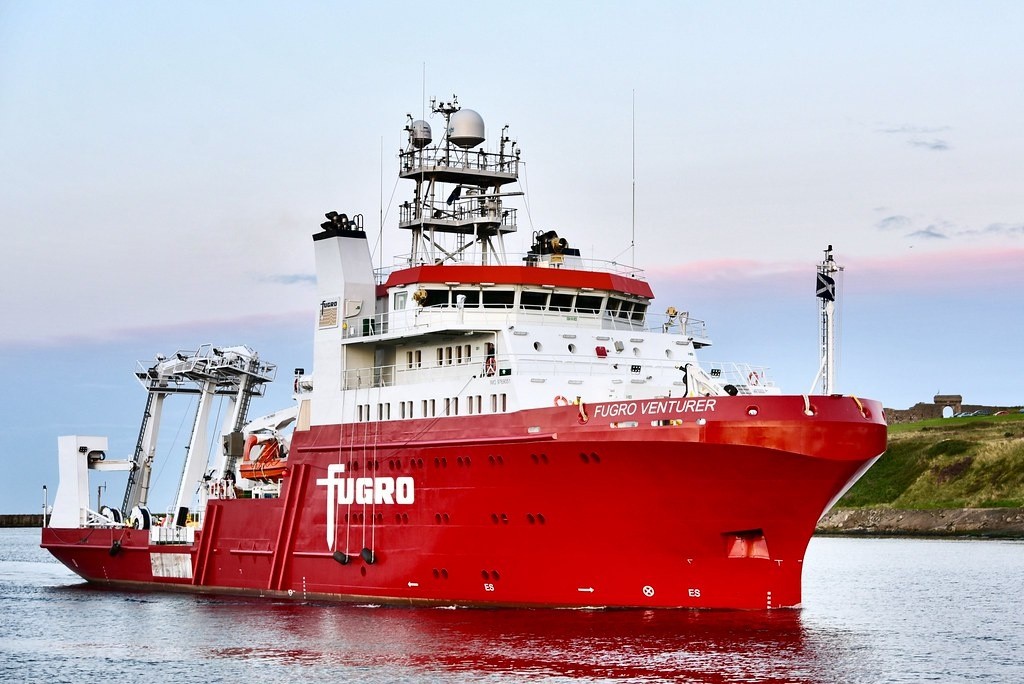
<box><xmin>953</xmin><ymin>412</ymin><xmax>962</xmax><ymax>417</ymax></box>
<box><xmin>992</xmin><ymin>411</ymin><xmax>1010</xmax><ymax>417</ymax></box>
<box><xmin>957</xmin><ymin>412</ymin><xmax>972</xmax><ymax>417</ymax></box>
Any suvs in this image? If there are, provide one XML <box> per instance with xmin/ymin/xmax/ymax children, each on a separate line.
<box><xmin>971</xmin><ymin>409</ymin><xmax>990</xmax><ymax>417</ymax></box>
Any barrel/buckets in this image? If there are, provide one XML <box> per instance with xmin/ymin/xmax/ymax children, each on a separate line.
<box><xmin>363</xmin><ymin>319</ymin><xmax>375</xmax><ymax>336</ymax></box>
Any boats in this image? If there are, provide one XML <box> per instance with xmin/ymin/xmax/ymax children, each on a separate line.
<box><xmin>38</xmin><ymin>61</ymin><xmax>888</xmax><ymax>613</ymax></box>
<box><xmin>239</xmin><ymin>430</ymin><xmax>289</xmax><ymax>480</ymax></box>
<box><xmin>211</xmin><ymin>345</ymin><xmax>259</xmax><ymax>362</ymax></box>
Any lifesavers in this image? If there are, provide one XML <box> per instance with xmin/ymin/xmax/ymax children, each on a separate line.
<box><xmin>486</xmin><ymin>358</ymin><xmax>497</xmax><ymax>374</ymax></box>
<box><xmin>748</xmin><ymin>370</ymin><xmax>759</xmax><ymax>386</ymax></box>
<box><xmin>553</xmin><ymin>395</ymin><xmax>567</xmax><ymax>406</ymax></box>
